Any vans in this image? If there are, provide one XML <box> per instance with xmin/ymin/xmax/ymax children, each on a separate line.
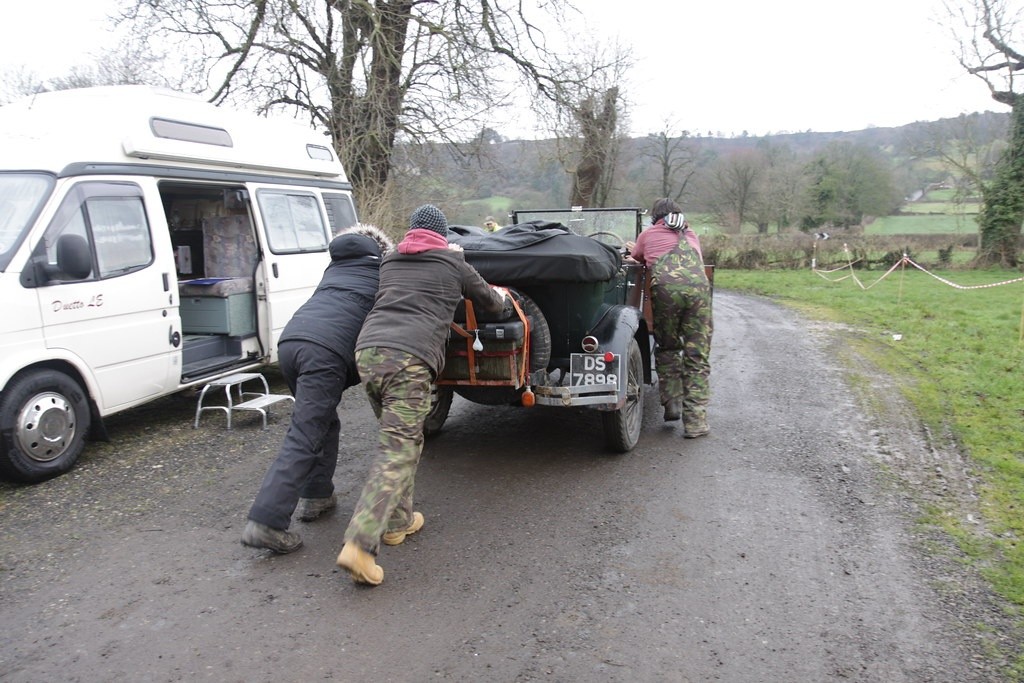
<box><xmin>0</xmin><ymin>84</ymin><xmax>361</xmax><ymax>487</ymax></box>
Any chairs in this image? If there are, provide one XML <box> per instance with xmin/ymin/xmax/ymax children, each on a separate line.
<box><xmin>177</xmin><ymin>215</ymin><xmax>259</xmax><ymax>336</ymax></box>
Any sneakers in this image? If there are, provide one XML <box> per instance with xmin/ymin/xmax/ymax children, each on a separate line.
<box><xmin>241</xmin><ymin>519</ymin><xmax>303</xmax><ymax>553</ymax></box>
<box><xmin>335</xmin><ymin>541</ymin><xmax>384</xmax><ymax>586</ymax></box>
<box><xmin>294</xmin><ymin>492</ymin><xmax>338</xmax><ymax>521</ymax></box>
<box><xmin>383</xmin><ymin>512</ymin><xmax>425</xmax><ymax>545</ymax></box>
<box><xmin>663</xmin><ymin>402</ymin><xmax>680</xmax><ymax>421</ymax></box>
<box><xmin>683</xmin><ymin>423</ymin><xmax>711</xmax><ymax>438</ymax></box>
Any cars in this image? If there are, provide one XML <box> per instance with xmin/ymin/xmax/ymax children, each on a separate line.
<box><xmin>424</xmin><ymin>206</ymin><xmax>716</xmax><ymax>455</ymax></box>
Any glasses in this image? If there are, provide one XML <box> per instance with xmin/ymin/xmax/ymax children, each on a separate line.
<box><xmin>485</xmin><ymin>222</ymin><xmax>493</xmax><ymax>225</ymax></box>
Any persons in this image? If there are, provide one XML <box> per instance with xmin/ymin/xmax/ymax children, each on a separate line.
<box><xmin>483</xmin><ymin>216</ymin><xmax>503</xmax><ymax>233</ymax></box>
<box><xmin>241</xmin><ymin>223</ymin><xmax>393</xmax><ymax>554</ymax></box>
<box><xmin>336</xmin><ymin>207</ymin><xmax>507</xmax><ymax>585</ymax></box>
<box><xmin>625</xmin><ymin>197</ymin><xmax>714</xmax><ymax>437</ymax></box>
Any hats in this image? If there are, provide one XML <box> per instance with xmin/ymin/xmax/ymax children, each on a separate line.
<box><xmin>409</xmin><ymin>204</ymin><xmax>449</xmax><ymax>238</ymax></box>
<box><xmin>651</xmin><ymin>198</ymin><xmax>683</xmax><ymax>224</ymax></box>
